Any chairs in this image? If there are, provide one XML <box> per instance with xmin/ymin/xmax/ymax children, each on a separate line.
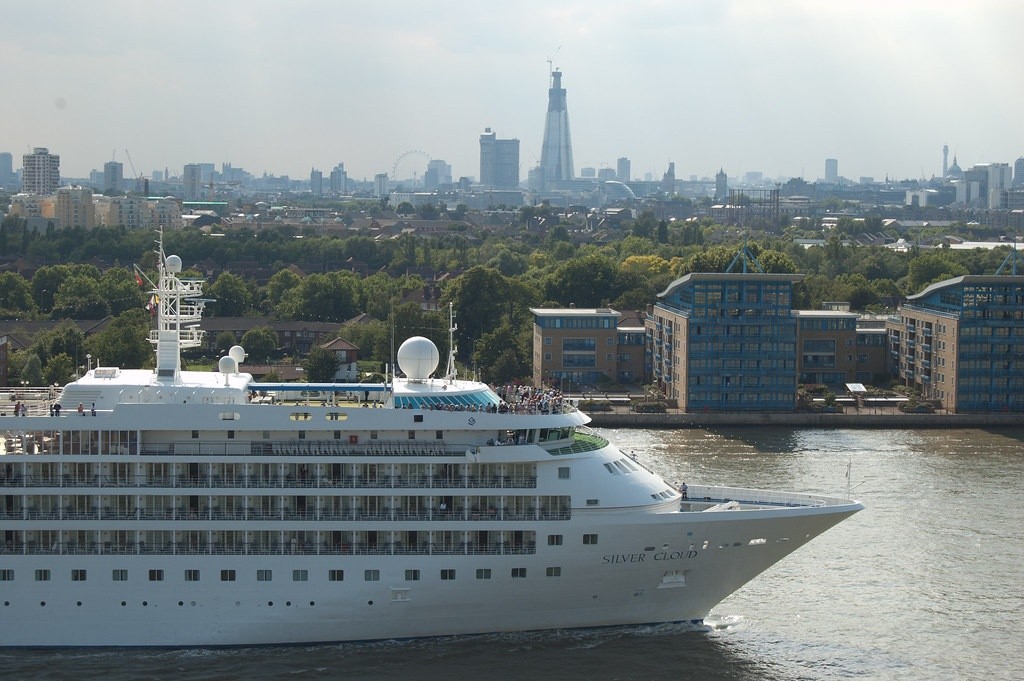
<box><xmin>0</xmin><ymin>474</ymin><xmax>569</xmax><ymax>555</ymax></box>
<box><xmin>272</xmin><ymin>439</ymin><xmax>447</xmax><ymax>457</ymax></box>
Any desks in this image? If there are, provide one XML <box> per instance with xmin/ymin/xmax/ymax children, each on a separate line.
<box><xmin>250</xmin><ymin>395</ymin><xmax>272</xmax><ymax>404</ymax></box>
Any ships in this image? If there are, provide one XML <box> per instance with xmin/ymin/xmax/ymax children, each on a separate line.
<box><xmin>0</xmin><ymin>225</ymin><xmax>863</xmax><ymax>650</ymax></box>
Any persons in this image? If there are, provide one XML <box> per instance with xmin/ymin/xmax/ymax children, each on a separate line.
<box><xmin>91</xmin><ymin>403</ymin><xmax>96</xmax><ymax>416</ymax></box>
<box><xmin>302</xmin><ymin>403</ymin><xmax>310</xmax><ymax>420</ymax></box>
<box><xmin>403</xmin><ymin>384</ymin><xmax>564</xmax><ymax>414</ymax></box>
<box><xmin>681</xmin><ymin>482</ymin><xmax>688</xmax><ymax>500</ymax></box>
<box><xmin>77</xmin><ymin>403</ymin><xmax>84</xmax><ymax>416</ymax></box>
<box><xmin>21</xmin><ymin>404</ymin><xmax>27</xmax><ymax>416</ymax></box>
<box><xmin>50</xmin><ymin>403</ymin><xmax>61</xmax><ymax>416</ymax></box>
<box><xmin>294</xmin><ymin>402</ymin><xmax>301</xmax><ymax>420</ymax></box>
<box><xmin>329</xmin><ymin>402</ymin><xmax>338</xmax><ymax>420</ymax></box>
<box><xmin>15</xmin><ymin>402</ymin><xmax>20</xmax><ymax>416</ymax></box>
<box><xmin>487</xmin><ymin>434</ymin><xmax>525</xmax><ymax>445</ymax></box>
<box><xmin>363</xmin><ymin>401</ymin><xmax>382</xmax><ymax>409</ymax></box>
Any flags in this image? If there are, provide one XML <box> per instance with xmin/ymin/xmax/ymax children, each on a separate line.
<box><xmin>145</xmin><ymin>296</ymin><xmax>156</xmax><ymax>310</ymax></box>
<box><xmin>135</xmin><ymin>272</ymin><xmax>143</xmax><ymax>287</ymax></box>
